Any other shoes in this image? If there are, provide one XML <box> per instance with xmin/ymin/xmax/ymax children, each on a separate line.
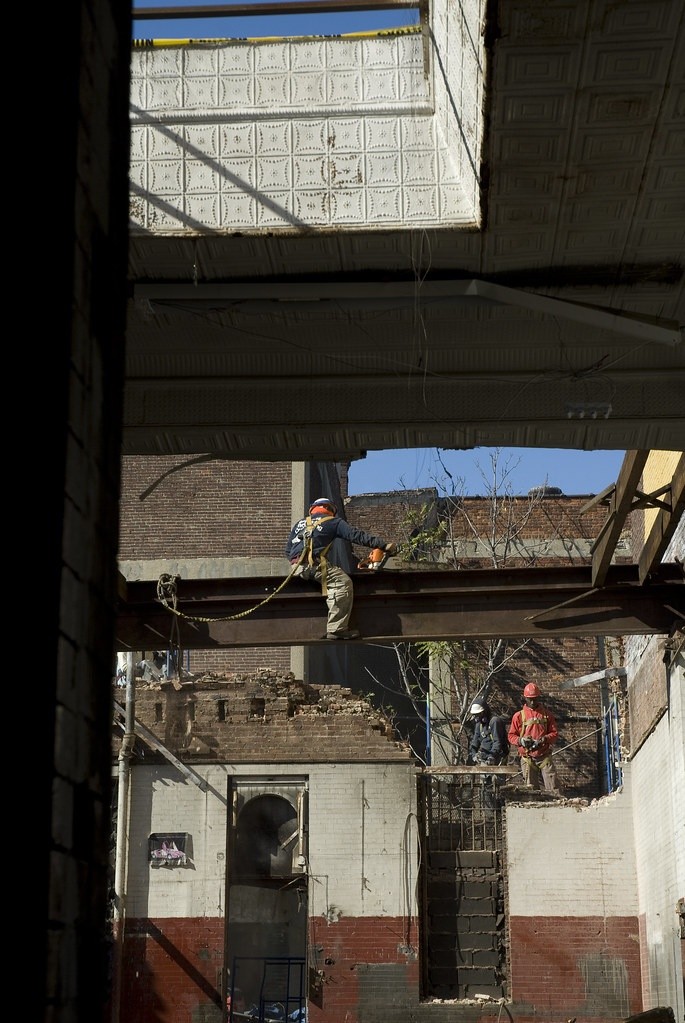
<box><xmin>327</xmin><ymin>629</ymin><xmax>359</xmax><ymax>639</ymax></box>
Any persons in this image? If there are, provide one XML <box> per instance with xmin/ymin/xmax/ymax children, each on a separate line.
<box><xmin>284</xmin><ymin>498</ymin><xmax>396</xmax><ymax>640</ymax></box>
<box><xmin>507</xmin><ymin>683</ymin><xmax>559</xmax><ymax>792</ymax></box>
<box><xmin>469</xmin><ymin>697</ymin><xmax>508</xmax><ymax>783</ymax></box>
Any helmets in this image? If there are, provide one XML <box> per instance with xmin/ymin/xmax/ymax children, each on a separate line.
<box><xmin>524</xmin><ymin>682</ymin><xmax>541</xmax><ymax>697</ymax></box>
<box><xmin>470</xmin><ymin>704</ymin><xmax>483</xmax><ymax>714</ymax></box>
<box><xmin>309</xmin><ymin>497</ymin><xmax>338</xmax><ymax>516</ymax></box>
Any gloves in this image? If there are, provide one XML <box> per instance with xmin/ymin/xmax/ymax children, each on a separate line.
<box><xmin>484</xmin><ymin>756</ymin><xmax>495</xmax><ymax>766</ymax></box>
<box><xmin>521</xmin><ymin>735</ymin><xmax>542</xmax><ymax>751</ymax></box>
